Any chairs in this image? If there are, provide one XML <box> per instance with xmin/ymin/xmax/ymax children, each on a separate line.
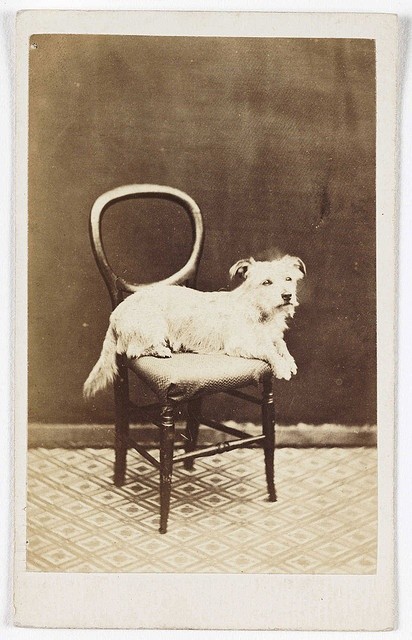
<box><xmin>89</xmin><ymin>181</ymin><xmax>277</xmax><ymax>534</ymax></box>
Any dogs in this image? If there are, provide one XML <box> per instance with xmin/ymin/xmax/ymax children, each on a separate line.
<box><xmin>83</xmin><ymin>254</ymin><xmax>306</xmax><ymax>403</ymax></box>
